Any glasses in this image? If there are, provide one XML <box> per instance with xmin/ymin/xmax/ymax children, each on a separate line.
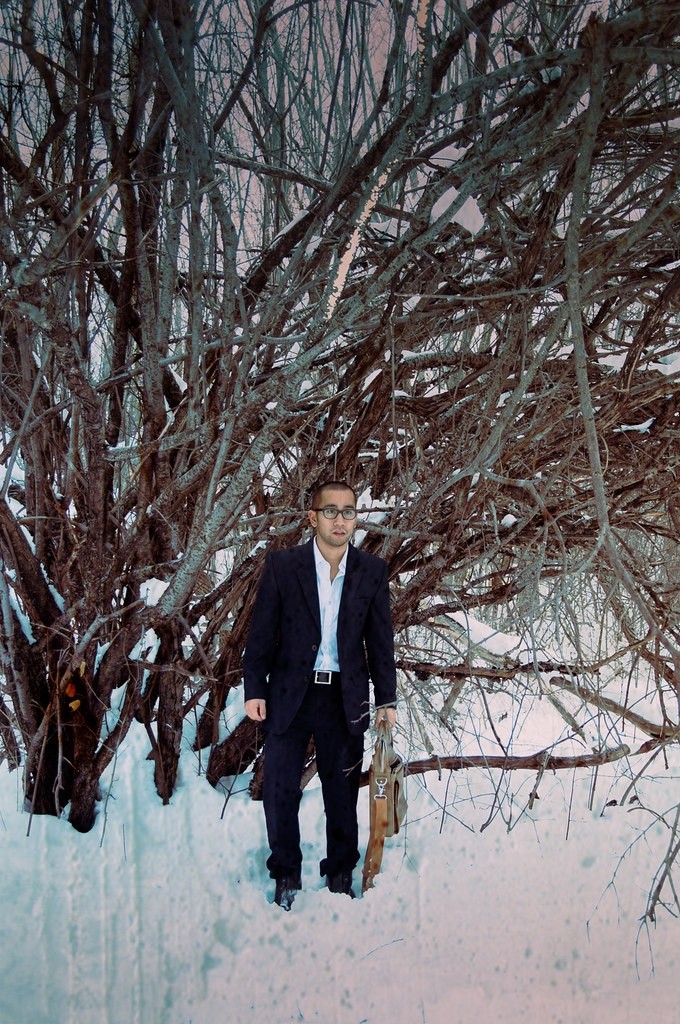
<box><xmin>314</xmin><ymin>506</ymin><xmax>358</xmax><ymax>520</ymax></box>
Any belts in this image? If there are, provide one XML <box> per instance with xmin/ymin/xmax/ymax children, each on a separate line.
<box><xmin>306</xmin><ymin>668</ymin><xmax>340</xmax><ymax>685</ymax></box>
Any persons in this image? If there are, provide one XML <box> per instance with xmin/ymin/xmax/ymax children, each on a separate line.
<box><xmin>243</xmin><ymin>481</ymin><xmax>396</xmax><ymax>911</ymax></box>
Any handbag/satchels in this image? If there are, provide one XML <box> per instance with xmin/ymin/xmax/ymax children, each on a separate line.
<box><xmin>361</xmin><ymin>719</ymin><xmax>408</xmax><ymax>837</ymax></box>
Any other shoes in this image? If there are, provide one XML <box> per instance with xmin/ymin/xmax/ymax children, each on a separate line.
<box><xmin>274</xmin><ymin>878</ymin><xmax>301</xmax><ymax>909</ymax></box>
<box><xmin>323</xmin><ymin>871</ymin><xmax>358</xmax><ymax>904</ymax></box>
